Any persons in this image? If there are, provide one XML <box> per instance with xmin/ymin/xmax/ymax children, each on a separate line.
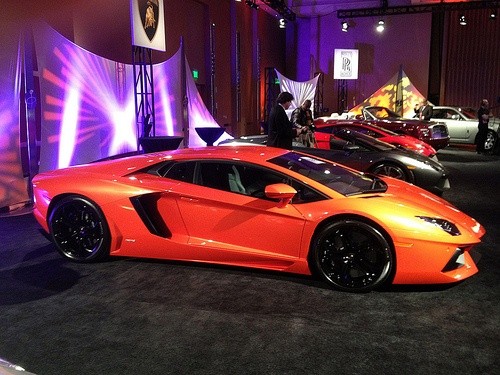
<box><xmin>414</xmin><ymin>99</ymin><xmax>433</xmax><ymax>121</ymax></box>
<box><xmin>266</xmin><ymin>91</ymin><xmax>307</xmax><ymax>150</ymax></box>
<box><xmin>290</xmin><ymin>99</ymin><xmax>315</xmax><ymax>148</ymax></box>
<box><xmin>477</xmin><ymin>99</ymin><xmax>494</xmax><ymax>156</ymax></box>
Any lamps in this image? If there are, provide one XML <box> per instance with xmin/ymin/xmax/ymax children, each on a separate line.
<box><xmin>459</xmin><ymin>11</ymin><xmax>468</xmax><ymax>26</ymax></box>
<box><xmin>245</xmin><ymin>0</ymin><xmax>260</xmax><ymax>10</ymax></box>
<box><xmin>489</xmin><ymin>8</ymin><xmax>498</xmax><ymax>22</ymax></box>
<box><xmin>340</xmin><ymin>18</ymin><xmax>351</xmax><ymax>33</ymax></box>
<box><xmin>378</xmin><ymin>16</ymin><xmax>385</xmax><ymax>25</ymax></box>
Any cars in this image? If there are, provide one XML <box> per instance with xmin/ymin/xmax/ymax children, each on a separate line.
<box><xmin>406</xmin><ymin>106</ymin><xmax>500</xmax><ymax>154</ymax></box>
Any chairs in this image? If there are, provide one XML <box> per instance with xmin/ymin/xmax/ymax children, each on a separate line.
<box><xmin>227</xmin><ymin>165</ymin><xmax>260</xmax><ymax>195</ymax></box>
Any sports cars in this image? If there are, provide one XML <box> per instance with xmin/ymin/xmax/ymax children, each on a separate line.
<box><xmin>311</xmin><ymin>119</ymin><xmax>440</xmax><ymax>163</ymax></box>
<box><xmin>216</xmin><ymin>128</ymin><xmax>451</xmax><ymax>201</ymax></box>
<box><xmin>312</xmin><ymin>105</ymin><xmax>449</xmax><ymax>154</ymax></box>
<box><xmin>31</xmin><ymin>145</ymin><xmax>486</xmax><ymax>293</ymax></box>
